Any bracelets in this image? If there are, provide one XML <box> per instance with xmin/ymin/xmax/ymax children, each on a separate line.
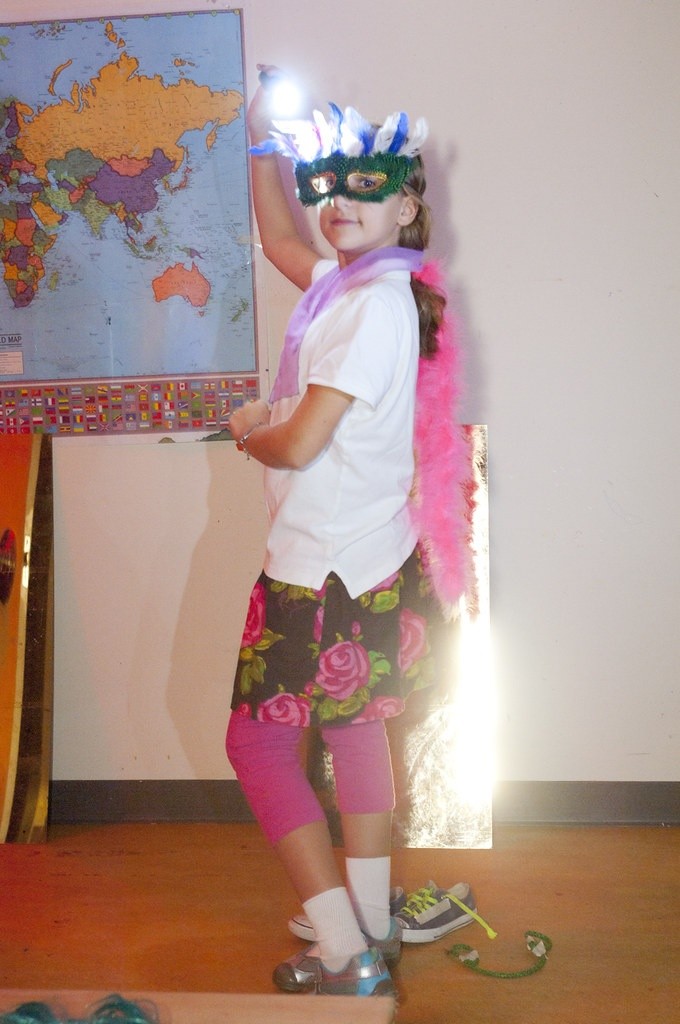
<box><xmin>239</xmin><ymin>422</ymin><xmax>269</xmax><ymax>460</ymax></box>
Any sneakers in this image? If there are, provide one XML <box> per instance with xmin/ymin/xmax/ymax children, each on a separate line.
<box><xmin>288</xmin><ymin>886</ymin><xmax>407</xmax><ymax>942</ymax></box>
<box><xmin>394</xmin><ymin>879</ymin><xmax>497</xmax><ymax>943</ymax></box>
<box><xmin>272</xmin><ymin>917</ymin><xmax>403</xmax><ymax>992</ymax></box>
<box><xmin>295</xmin><ymin>946</ymin><xmax>400</xmax><ymax>999</ymax></box>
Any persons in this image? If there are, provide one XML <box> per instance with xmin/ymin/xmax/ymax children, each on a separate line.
<box><xmin>223</xmin><ymin>111</ymin><xmax>470</xmax><ymax>999</ymax></box>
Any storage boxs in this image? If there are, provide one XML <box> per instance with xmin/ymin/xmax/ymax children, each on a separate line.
<box><xmin>0</xmin><ymin>983</ymin><xmax>395</xmax><ymax>1024</ymax></box>
